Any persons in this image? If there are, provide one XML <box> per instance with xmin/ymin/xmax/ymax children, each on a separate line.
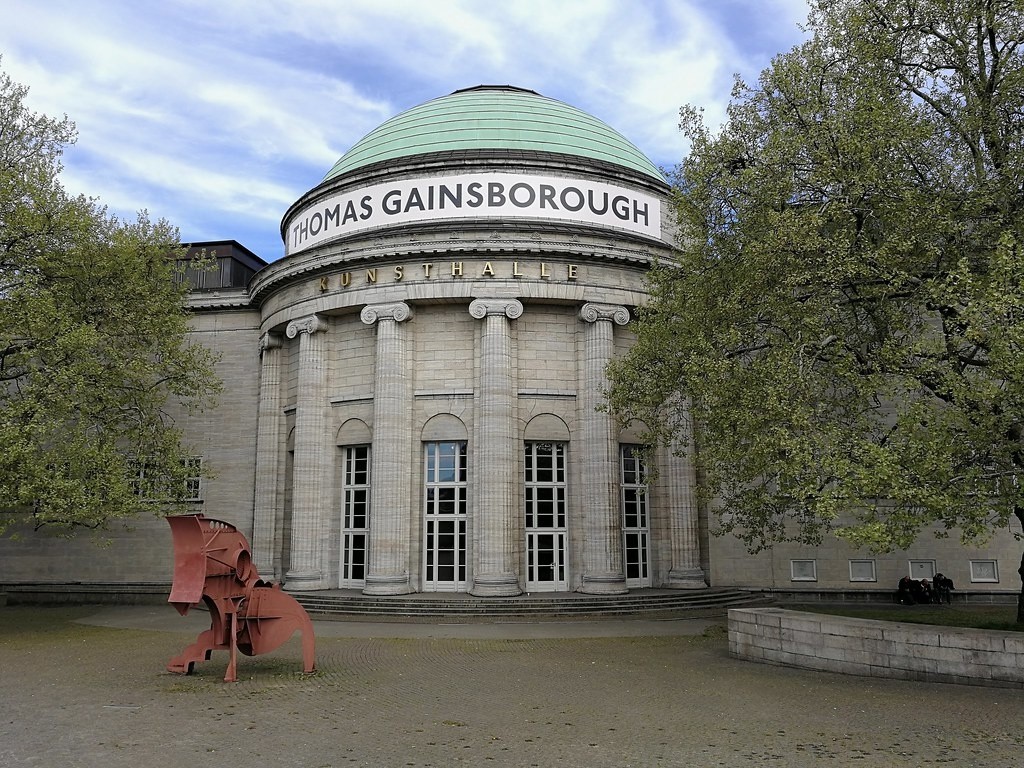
<box><xmin>898</xmin><ymin>573</ymin><xmax>952</xmax><ymax>605</ymax></box>
